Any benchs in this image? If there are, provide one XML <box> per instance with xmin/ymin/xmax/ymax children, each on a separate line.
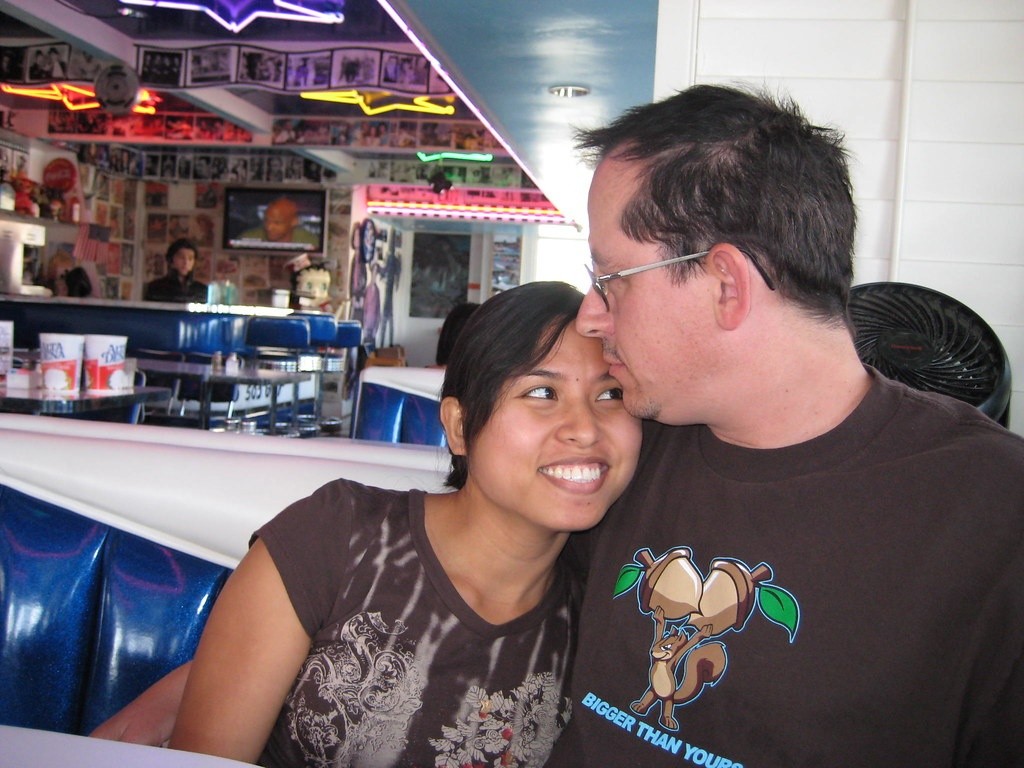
<box><xmin>0</xmin><ymin>414</ymin><xmax>459</xmax><ymax>736</ymax></box>
<box><xmin>350</xmin><ymin>367</ymin><xmax>453</xmax><ymax>450</ymax></box>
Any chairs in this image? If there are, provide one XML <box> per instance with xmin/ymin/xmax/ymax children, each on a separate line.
<box><xmin>231</xmin><ymin>309</ymin><xmax>363</xmax><ymax>437</ymax></box>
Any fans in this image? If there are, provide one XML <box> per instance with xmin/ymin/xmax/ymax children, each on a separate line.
<box><xmin>845</xmin><ymin>282</ymin><xmax>1012</xmax><ymax>431</ymax></box>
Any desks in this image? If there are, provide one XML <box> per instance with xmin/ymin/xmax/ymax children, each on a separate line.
<box><xmin>0</xmin><ymin>387</ymin><xmax>171</xmax><ymax>420</ymax></box>
<box><xmin>134</xmin><ymin>358</ymin><xmax>312</xmax><ymax>433</ymax></box>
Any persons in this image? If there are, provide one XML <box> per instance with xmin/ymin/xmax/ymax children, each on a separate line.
<box><xmin>26</xmin><ymin>42</ymin><xmax>432</xmax><ymax>91</ymax></box>
<box><xmin>236</xmin><ymin>196</ymin><xmax>322</xmax><ymax>250</ymax></box>
<box><xmin>90</xmin><ymin>82</ymin><xmax>1024</xmax><ymax>768</ymax></box>
<box><xmin>170</xmin><ymin>281</ymin><xmax>644</xmax><ymax>768</ymax></box>
<box><xmin>51</xmin><ymin>107</ymin><xmax>511</xmax><ymax>151</ymax></box>
<box><xmin>91</xmin><ymin>147</ymin><xmax>522</xmax><ymax>185</ymax></box>
<box><xmin>142</xmin><ymin>235</ymin><xmax>208</xmax><ymax>303</ymax></box>
<box><xmin>286</xmin><ymin>253</ymin><xmax>334</xmax><ymax>314</ymax></box>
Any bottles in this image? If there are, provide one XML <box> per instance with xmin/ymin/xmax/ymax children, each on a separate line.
<box><xmin>212</xmin><ymin>351</ymin><xmax>222</xmax><ymax>370</ymax></box>
<box><xmin>226</xmin><ymin>352</ymin><xmax>238</xmax><ymax>376</ymax></box>
<box><xmin>225</xmin><ymin>418</ymin><xmax>256</xmax><ymax>436</ymax></box>
<box><xmin>70</xmin><ymin>193</ymin><xmax>80</xmax><ymax>224</ymax></box>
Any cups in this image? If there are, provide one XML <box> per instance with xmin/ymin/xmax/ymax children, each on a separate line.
<box><xmin>40</xmin><ymin>334</ymin><xmax>128</xmax><ymax>394</ymax></box>
<box><xmin>207</xmin><ymin>283</ymin><xmax>221</xmax><ymax>304</ymax></box>
<box><xmin>272</xmin><ymin>289</ymin><xmax>290</xmax><ymax>308</ymax></box>
<box><xmin>226</xmin><ymin>287</ymin><xmax>239</xmax><ymax>305</ymax></box>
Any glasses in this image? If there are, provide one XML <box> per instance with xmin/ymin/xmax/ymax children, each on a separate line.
<box><xmin>584</xmin><ymin>248</ymin><xmax>776</xmax><ymax>312</ymax></box>
